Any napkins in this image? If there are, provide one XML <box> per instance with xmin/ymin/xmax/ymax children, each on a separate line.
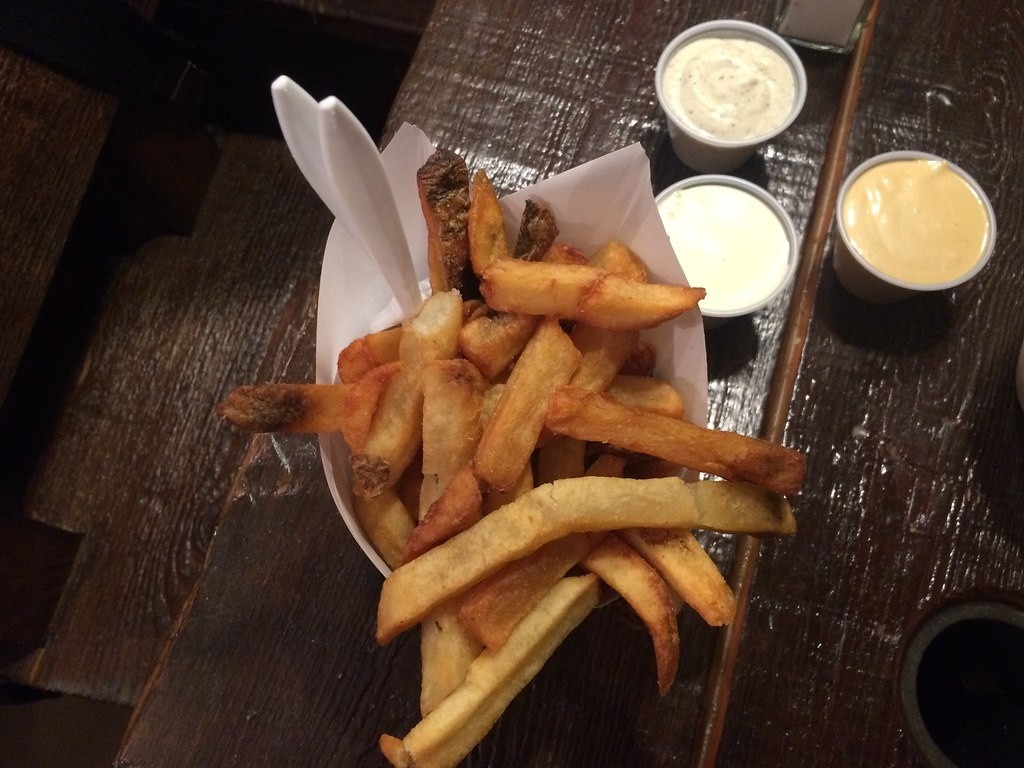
<box><xmin>314</xmin><ymin>121</ymin><xmax>709</xmax><ymax>578</ymax></box>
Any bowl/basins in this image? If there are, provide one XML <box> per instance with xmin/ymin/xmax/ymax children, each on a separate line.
<box><xmin>318</xmin><ymin>434</ymin><xmax>620</xmax><ymax>608</ymax></box>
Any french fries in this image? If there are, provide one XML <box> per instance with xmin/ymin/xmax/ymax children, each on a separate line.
<box><xmin>215</xmin><ymin>147</ymin><xmax>807</xmax><ymax>768</ymax></box>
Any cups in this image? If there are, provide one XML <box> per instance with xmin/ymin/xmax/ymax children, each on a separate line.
<box><xmin>655</xmin><ymin>19</ymin><xmax>808</xmax><ymax>172</ymax></box>
<box><xmin>652</xmin><ymin>175</ymin><xmax>799</xmax><ymax>327</ymax></box>
<box><xmin>832</xmin><ymin>150</ymin><xmax>996</xmax><ymax>305</ymax></box>
